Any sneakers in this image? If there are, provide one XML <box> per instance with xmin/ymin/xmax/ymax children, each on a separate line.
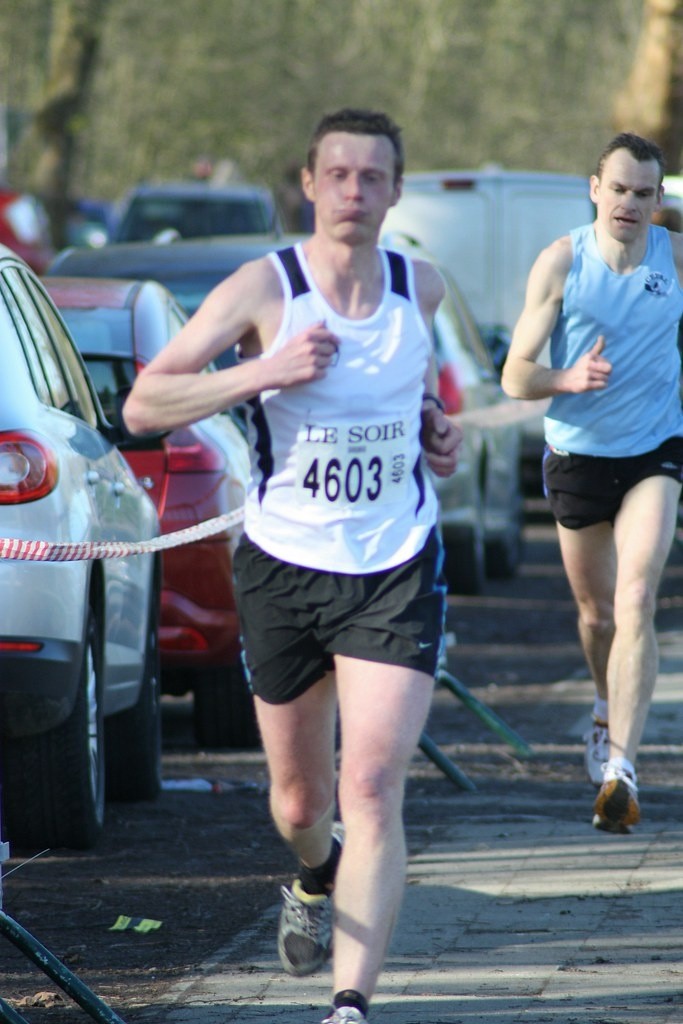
<box><xmin>321</xmin><ymin>995</ymin><xmax>368</xmax><ymax>1024</ymax></box>
<box><xmin>277</xmin><ymin>820</ymin><xmax>345</xmax><ymax>978</ymax></box>
<box><xmin>592</xmin><ymin>762</ymin><xmax>641</xmax><ymax>834</ymax></box>
<box><xmin>582</xmin><ymin>712</ymin><xmax>611</xmax><ymax>788</ymax></box>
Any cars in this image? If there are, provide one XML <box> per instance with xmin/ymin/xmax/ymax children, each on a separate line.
<box><xmin>1</xmin><ymin>168</ymin><xmax>682</xmax><ymax>590</ymax></box>
<box><xmin>34</xmin><ymin>273</ymin><xmax>271</xmax><ymax>751</ymax></box>
<box><xmin>0</xmin><ymin>241</ymin><xmax>176</xmax><ymax>851</ymax></box>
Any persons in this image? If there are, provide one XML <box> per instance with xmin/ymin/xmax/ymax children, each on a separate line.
<box><xmin>501</xmin><ymin>131</ymin><xmax>682</xmax><ymax>834</ymax></box>
<box><xmin>121</xmin><ymin>107</ymin><xmax>463</xmax><ymax>1024</ymax></box>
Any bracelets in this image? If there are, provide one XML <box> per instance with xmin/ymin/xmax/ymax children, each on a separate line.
<box><xmin>421</xmin><ymin>390</ymin><xmax>447</xmax><ymax>411</ymax></box>
